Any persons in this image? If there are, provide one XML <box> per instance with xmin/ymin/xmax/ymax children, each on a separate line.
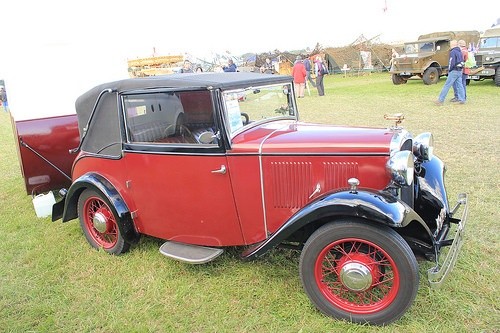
<box><xmin>303</xmin><ymin>54</ymin><xmax>317</xmax><ymax>89</ymax></box>
<box><xmin>292</xmin><ymin>56</ymin><xmax>307</xmax><ymax>98</ymax></box>
<box><xmin>450</xmin><ymin>40</ymin><xmax>470</xmax><ymax>102</ymax></box>
<box><xmin>221</xmin><ymin>59</ymin><xmax>237</xmax><ymax>72</ymax></box>
<box><xmin>389</xmin><ymin>48</ymin><xmax>399</xmax><ymax>71</ymax></box>
<box><xmin>433</xmin><ymin>40</ymin><xmax>466</xmax><ymax>104</ymax></box>
<box><xmin>196</xmin><ymin>67</ymin><xmax>203</xmax><ymax>72</ymax></box>
<box><xmin>177</xmin><ymin>60</ymin><xmax>194</xmax><ymax>74</ymax></box>
<box><xmin>0</xmin><ymin>87</ymin><xmax>8</xmax><ymax>112</ymax></box>
<box><xmin>314</xmin><ymin>54</ymin><xmax>325</xmax><ymax>96</ymax></box>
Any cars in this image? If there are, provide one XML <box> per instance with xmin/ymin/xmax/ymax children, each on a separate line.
<box><xmin>15</xmin><ymin>72</ymin><xmax>469</xmax><ymax>325</ymax></box>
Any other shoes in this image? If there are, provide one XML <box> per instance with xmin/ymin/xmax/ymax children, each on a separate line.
<box><xmin>431</xmin><ymin>97</ymin><xmax>465</xmax><ymax>105</ymax></box>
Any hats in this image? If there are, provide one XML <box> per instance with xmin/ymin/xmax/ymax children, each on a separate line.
<box><xmin>296</xmin><ymin>56</ymin><xmax>301</xmax><ymax>59</ymax></box>
<box><xmin>458</xmin><ymin>40</ymin><xmax>466</xmax><ymax>47</ymax></box>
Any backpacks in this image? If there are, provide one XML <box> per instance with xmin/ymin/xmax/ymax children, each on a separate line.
<box><xmin>462</xmin><ymin>50</ymin><xmax>476</xmax><ymax>68</ymax></box>
<box><xmin>320</xmin><ymin>61</ymin><xmax>328</xmax><ymax>74</ymax></box>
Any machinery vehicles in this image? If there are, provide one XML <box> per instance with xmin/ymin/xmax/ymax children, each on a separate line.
<box><xmin>389</xmin><ymin>31</ymin><xmax>480</xmax><ymax>85</ymax></box>
<box><xmin>466</xmin><ymin>27</ymin><xmax>500</xmax><ymax>86</ymax></box>
